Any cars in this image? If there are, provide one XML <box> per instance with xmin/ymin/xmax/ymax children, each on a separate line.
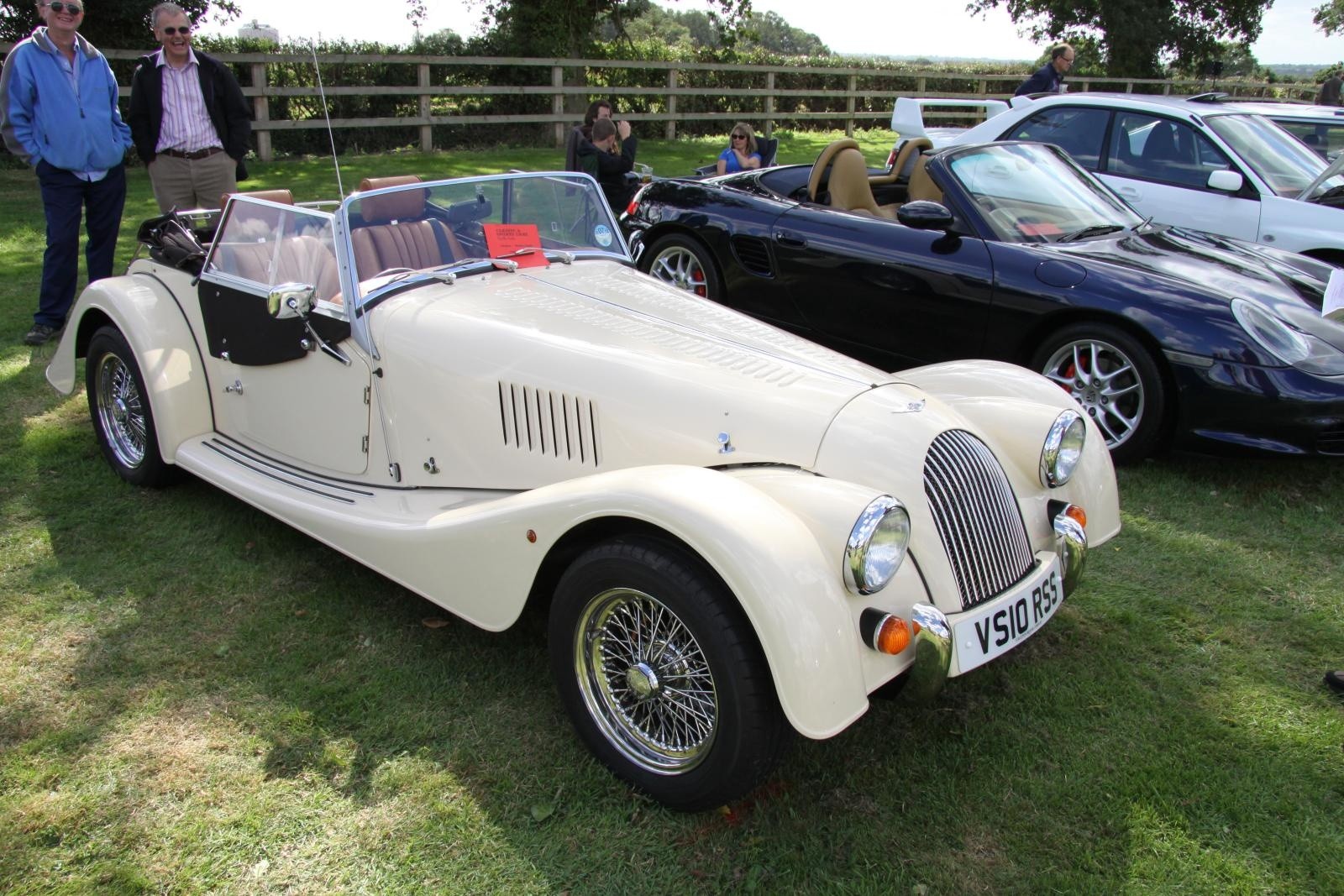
<box><xmin>43</xmin><ymin>169</ymin><xmax>1127</xmax><ymax>814</ymax></box>
<box><xmin>883</xmin><ymin>88</ymin><xmax>1344</xmax><ymax>273</ymax></box>
<box><xmin>613</xmin><ymin>137</ymin><xmax>1344</xmax><ymax>478</ymax></box>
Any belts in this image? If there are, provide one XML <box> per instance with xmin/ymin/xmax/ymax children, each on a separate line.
<box><xmin>161</xmin><ymin>147</ymin><xmax>221</xmax><ymax>161</ymax></box>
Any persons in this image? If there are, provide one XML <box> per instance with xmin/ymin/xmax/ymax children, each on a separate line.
<box><xmin>1014</xmin><ymin>42</ymin><xmax>1074</xmax><ymax>96</ymax></box>
<box><xmin>589</xmin><ymin>117</ymin><xmax>637</xmax><ymax>215</ymax></box>
<box><xmin>717</xmin><ymin>122</ymin><xmax>761</xmax><ymax>177</ymax></box>
<box><xmin>127</xmin><ymin>3</ymin><xmax>253</xmax><ymax>216</ymax></box>
<box><xmin>0</xmin><ymin>0</ymin><xmax>134</xmax><ymax>345</ymax></box>
<box><xmin>575</xmin><ymin>99</ymin><xmax>619</xmax><ymax>154</ymax></box>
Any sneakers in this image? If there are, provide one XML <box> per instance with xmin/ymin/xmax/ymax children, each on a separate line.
<box><xmin>25</xmin><ymin>322</ymin><xmax>62</xmax><ymax>344</ymax></box>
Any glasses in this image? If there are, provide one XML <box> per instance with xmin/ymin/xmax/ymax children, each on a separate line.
<box><xmin>732</xmin><ymin>134</ymin><xmax>748</xmax><ymax>139</ymax></box>
<box><xmin>1055</xmin><ymin>54</ymin><xmax>1074</xmax><ymax>64</ymax></box>
<box><xmin>156</xmin><ymin>27</ymin><xmax>189</xmax><ymax>35</ymax></box>
<box><xmin>41</xmin><ymin>1</ymin><xmax>83</xmax><ymax>16</ymax></box>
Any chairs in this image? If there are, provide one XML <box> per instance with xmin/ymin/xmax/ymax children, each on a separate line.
<box><xmin>1303</xmin><ymin>134</ymin><xmax>1328</xmax><ymax>160</ymax></box>
<box><xmin>1143</xmin><ymin>122</ymin><xmax>1181</xmax><ymax>159</ymax></box>
<box><xmin>212</xmin><ymin>190</ymin><xmax>342</xmax><ymax>302</ymax></box>
<box><xmin>573</xmin><ymin>123</ymin><xmax>778</xmax><ymax>242</ymax></box>
<box><xmin>351</xmin><ymin>175</ymin><xmax>467</xmax><ymax>282</ymax></box>
<box><xmin>1088</xmin><ymin>127</ymin><xmax>1131</xmax><ymax>160</ymax></box>
<box><xmin>827</xmin><ymin>148</ymin><xmax>883</xmax><ymax>217</ymax></box>
<box><xmin>907</xmin><ymin>155</ymin><xmax>943</xmax><ymax>204</ymax></box>
